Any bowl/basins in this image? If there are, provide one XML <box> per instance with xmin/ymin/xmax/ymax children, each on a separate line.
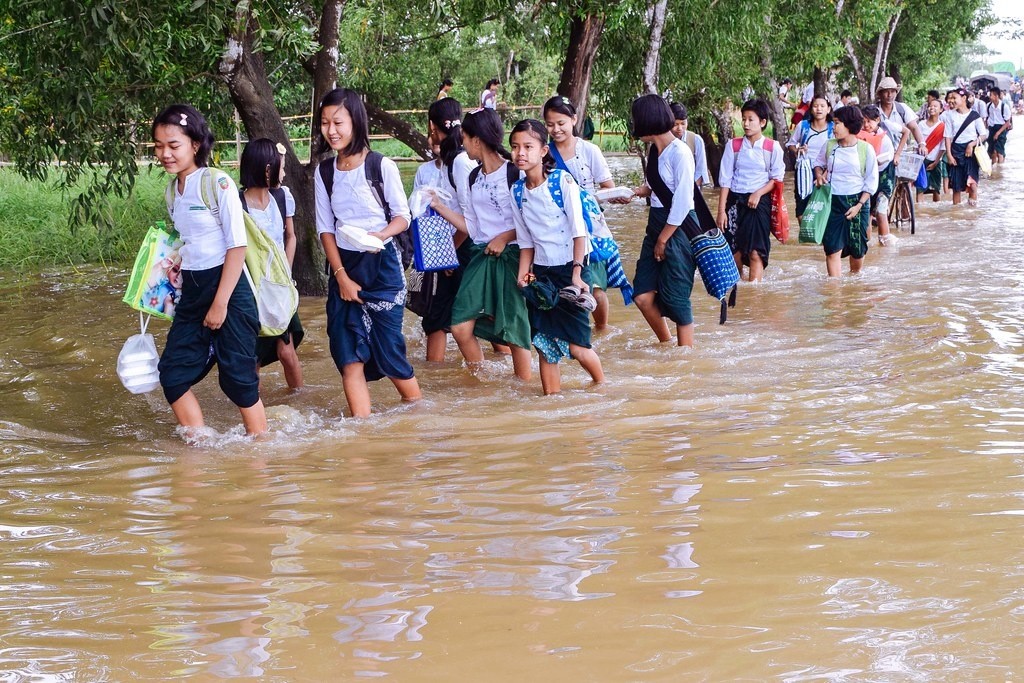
<box><xmin>338</xmin><ymin>225</ymin><xmax>386</xmax><ymax>253</ymax></box>
<box><xmin>877</xmin><ymin>151</ymin><xmax>890</xmax><ymax>163</ymax></box>
<box><xmin>594</xmin><ymin>185</ymin><xmax>634</xmax><ymax>202</ymax></box>
<box><xmin>408</xmin><ymin>191</ymin><xmax>422</xmax><ymax>220</ymax></box>
<box><xmin>119</xmin><ymin>351</ymin><xmax>160</xmax><ymax>394</ymax></box>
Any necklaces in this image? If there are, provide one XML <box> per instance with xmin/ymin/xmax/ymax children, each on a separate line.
<box><xmin>339</xmin><ymin>151</ymin><xmax>364</xmax><ymax>167</ymax></box>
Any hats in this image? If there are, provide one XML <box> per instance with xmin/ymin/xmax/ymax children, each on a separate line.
<box><xmin>876</xmin><ymin>76</ymin><xmax>903</xmax><ymax>94</ymax></box>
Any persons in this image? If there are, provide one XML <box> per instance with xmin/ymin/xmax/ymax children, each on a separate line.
<box><xmin>152</xmin><ymin>106</ymin><xmax>269</xmax><ymax>439</ymax></box>
<box><xmin>240</xmin><ymin>64</ymin><xmax>1024</xmax><ymax>421</ymax></box>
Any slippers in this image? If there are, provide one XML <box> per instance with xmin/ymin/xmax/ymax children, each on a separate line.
<box><xmin>559</xmin><ymin>285</ymin><xmax>598</xmax><ymax>312</ymax></box>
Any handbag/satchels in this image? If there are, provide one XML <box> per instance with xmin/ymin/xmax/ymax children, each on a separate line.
<box><xmin>769</xmin><ymin>178</ymin><xmax>789</xmax><ymax>244</ymax></box>
<box><xmin>798</xmin><ymin>183</ymin><xmax>832</xmax><ymax>245</ymax></box>
<box><xmin>795</xmin><ymin>149</ymin><xmax>814</xmax><ymax>199</ymax></box>
<box><xmin>914</xmin><ymin>150</ymin><xmax>929</xmax><ymax>190</ymax></box>
<box><xmin>790</xmin><ymin>100</ymin><xmax>809</xmax><ymax>130</ymax></box>
<box><xmin>411</xmin><ymin>208</ymin><xmax>461</xmax><ymax>271</ymax></box>
<box><xmin>116</xmin><ymin>312</ymin><xmax>161</xmax><ymax>395</ymax></box>
<box><xmin>122</xmin><ymin>219</ymin><xmax>183</xmax><ymax>321</ymax></box>
<box><xmin>646</xmin><ymin>142</ymin><xmax>740</xmax><ymax>325</ymax></box>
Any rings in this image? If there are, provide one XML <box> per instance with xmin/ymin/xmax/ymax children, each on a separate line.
<box><xmin>656</xmin><ymin>256</ymin><xmax>660</xmax><ymax>259</ymax></box>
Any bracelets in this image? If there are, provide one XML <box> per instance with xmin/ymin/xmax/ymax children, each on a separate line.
<box><xmin>858</xmin><ymin>202</ymin><xmax>865</xmax><ymax>207</ymax></box>
<box><xmin>572</xmin><ymin>259</ymin><xmax>584</xmax><ymax>271</ymax></box>
<box><xmin>333</xmin><ymin>267</ymin><xmax>345</xmax><ymax>277</ymax></box>
<box><xmin>917</xmin><ymin>142</ymin><xmax>927</xmax><ymax>147</ymax></box>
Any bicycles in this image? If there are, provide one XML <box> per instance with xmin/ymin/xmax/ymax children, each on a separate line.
<box><xmin>887</xmin><ymin>147</ymin><xmax>930</xmax><ymax>234</ymax></box>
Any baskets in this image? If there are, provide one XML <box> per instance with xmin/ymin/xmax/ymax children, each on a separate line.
<box><xmin>895</xmin><ymin>152</ymin><xmax>925</xmax><ymax>182</ymax></box>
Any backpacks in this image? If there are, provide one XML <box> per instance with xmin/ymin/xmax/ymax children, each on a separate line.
<box><xmin>171</xmin><ymin>167</ymin><xmax>300</xmax><ymax>337</ymax></box>
<box><xmin>320</xmin><ymin>151</ymin><xmax>414</xmax><ymax>272</ymax></box>
<box><xmin>987</xmin><ymin>101</ymin><xmax>1013</xmax><ymax>130</ymax></box>
<box><xmin>512</xmin><ymin>170</ymin><xmax>618</xmax><ymax>262</ymax></box>
